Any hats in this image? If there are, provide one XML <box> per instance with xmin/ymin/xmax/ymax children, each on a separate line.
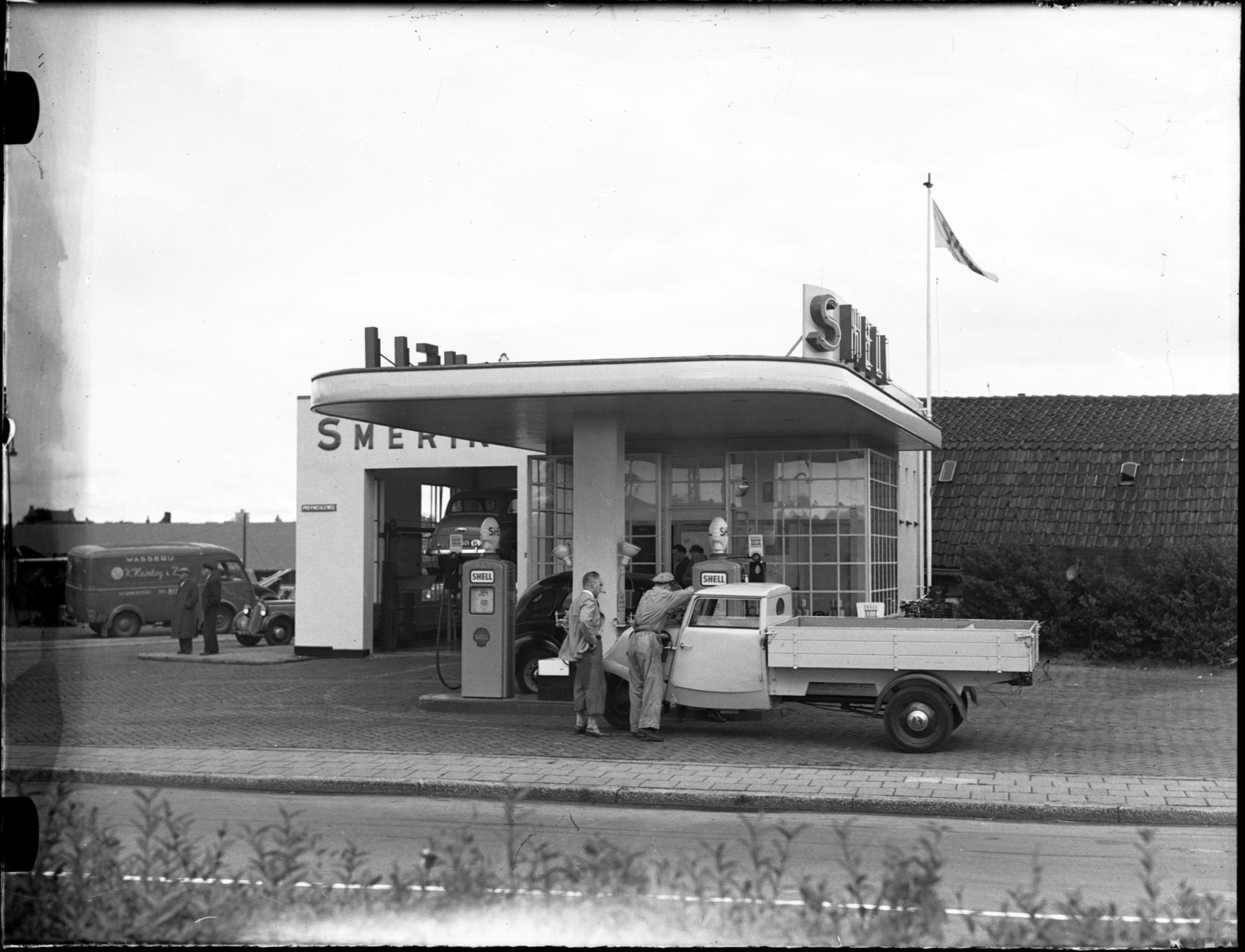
<box><xmin>652</xmin><ymin>572</ymin><xmax>675</xmax><ymax>584</ymax></box>
<box><xmin>176</xmin><ymin>567</ymin><xmax>190</xmax><ymax>573</ymax></box>
<box><xmin>203</xmin><ymin>563</ymin><xmax>215</xmax><ymax>570</ymax></box>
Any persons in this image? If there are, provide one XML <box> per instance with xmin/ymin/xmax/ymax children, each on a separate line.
<box><xmin>559</xmin><ymin>571</ymin><xmax>608</xmax><ymax>739</ymax></box>
<box><xmin>200</xmin><ymin>562</ymin><xmax>222</xmax><ymax>655</ymax></box>
<box><xmin>626</xmin><ymin>572</ymin><xmax>695</xmax><ymax>743</ymax></box>
<box><xmin>171</xmin><ymin>568</ymin><xmax>199</xmax><ymax>654</ymax></box>
<box><xmin>671</xmin><ymin>544</ymin><xmax>708</xmax><ymax>589</ymax></box>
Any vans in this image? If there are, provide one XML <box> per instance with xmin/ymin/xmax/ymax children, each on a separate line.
<box><xmin>59</xmin><ymin>540</ymin><xmax>255</xmax><ymax>639</ymax></box>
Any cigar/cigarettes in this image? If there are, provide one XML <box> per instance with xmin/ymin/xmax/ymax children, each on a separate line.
<box><xmin>599</xmin><ymin>591</ymin><xmax>606</xmax><ymax>594</ymax></box>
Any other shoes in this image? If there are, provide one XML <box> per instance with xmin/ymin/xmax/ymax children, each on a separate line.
<box><xmin>633</xmin><ymin>732</ymin><xmax>646</xmax><ymax>738</ymax></box>
<box><xmin>178</xmin><ymin>650</ymin><xmax>185</xmax><ymax>653</ymax></box>
<box><xmin>585</xmin><ymin>728</ymin><xmax>610</xmax><ymax>737</ymax></box>
<box><xmin>186</xmin><ymin>650</ymin><xmax>191</xmax><ymax>654</ymax></box>
<box><xmin>638</xmin><ymin>728</ymin><xmax>663</xmax><ymax>741</ymax></box>
<box><xmin>574</xmin><ymin>724</ymin><xmax>587</xmax><ymax>734</ymax></box>
<box><xmin>200</xmin><ymin>651</ymin><xmax>213</xmax><ymax>655</ymax></box>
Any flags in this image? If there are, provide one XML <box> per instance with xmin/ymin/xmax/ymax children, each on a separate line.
<box><xmin>932</xmin><ymin>198</ymin><xmax>999</xmax><ymax>282</ymax></box>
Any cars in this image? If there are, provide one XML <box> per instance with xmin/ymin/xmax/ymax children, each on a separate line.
<box><xmin>229</xmin><ymin>567</ymin><xmax>295</xmax><ymax>646</ymax></box>
<box><xmin>428</xmin><ymin>485</ymin><xmax>517</xmax><ymax>558</ymax></box>
<box><xmin>513</xmin><ymin>570</ymin><xmax>573</xmax><ymax>694</ymax></box>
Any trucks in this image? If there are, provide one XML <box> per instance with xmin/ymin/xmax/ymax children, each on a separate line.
<box><xmin>602</xmin><ymin>553</ymin><xmax>1040</xmax><ymax>754</ymax></box>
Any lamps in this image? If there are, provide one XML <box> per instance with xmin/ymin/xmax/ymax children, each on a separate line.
<box><xmin>618</xmin><ymin>541</ymin><xmax>641</xmax><ymax>566</ymax></box>
<box><xmin>551</xmin><ymin>542</ymin><xmax>573</xmax><ymax>567</ymax></box>
